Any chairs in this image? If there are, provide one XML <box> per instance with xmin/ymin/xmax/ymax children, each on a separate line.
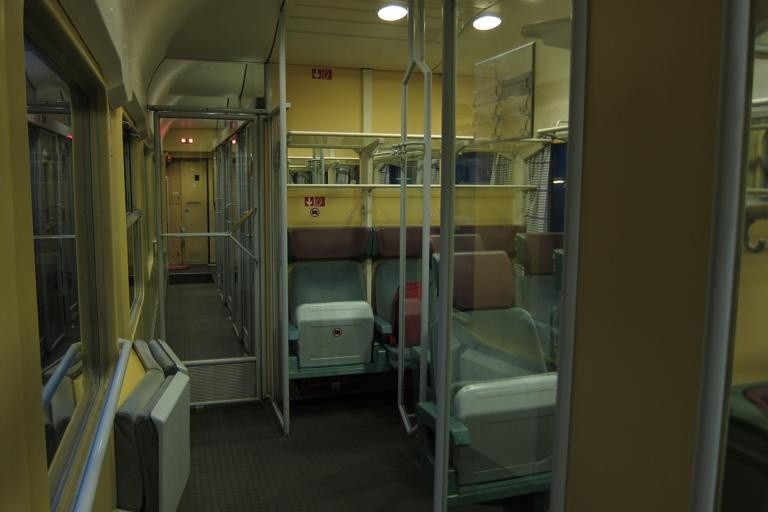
<box><xmin>373</xmin><ymin>225</ymin><xmax>565</xmax><ymax>512</ymax></box>
<box><xmin>288</xmin><ymin>226</ymin><xmax>391</xmax><ymax>399</ymax></box>
